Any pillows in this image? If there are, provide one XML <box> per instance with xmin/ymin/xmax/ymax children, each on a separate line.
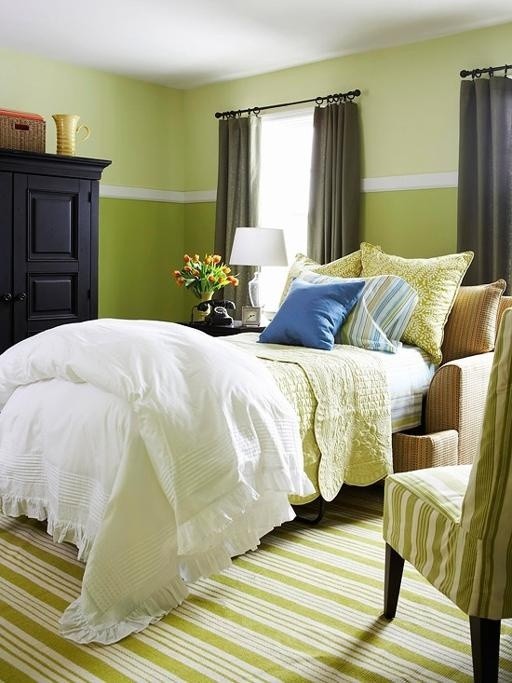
<box><xmin>278</xmin><ymin>253</ymin><xmax>363</xmax><ymax>307</ymax></box>
<box><xmin>441</xmin><ymin>277</ymin><xmax>506</xmax><ymax>365</ymax></box>
<box><xmin>360</xmin><ymin>242</ymin><xmax>475</xmax><ymax>364</ymax></box>
<box><xmin>288</xmin><ymin>269</ymin><xmax>418</xmax><ymax>350</ymax></box>
<box><xmin>258</xmin><ymin>277</ymin><xmax>367</xmax><ymax>350</ymax></box>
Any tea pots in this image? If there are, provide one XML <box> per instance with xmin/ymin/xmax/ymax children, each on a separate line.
<box><xmin>52</xmin><ymin>110</ymin><xmax>92</xmax><ymax>157</ymax></box>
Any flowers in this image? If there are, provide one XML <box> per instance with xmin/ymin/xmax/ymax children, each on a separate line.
<box><xmin>171</xmin><ymin>252</ymin><xmax>240</xmax><ymax>291</ymax></box>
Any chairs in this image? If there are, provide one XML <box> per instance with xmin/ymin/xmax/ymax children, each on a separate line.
<box><xmin>383</xmin><ymin>308</ymin><xmax>512</xmax><ymax>683</ymax></box>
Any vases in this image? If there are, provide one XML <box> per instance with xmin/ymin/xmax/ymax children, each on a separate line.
<box><xmin>192</xmin><ymin>289</ymin><xmax>215</xmax><ymax>322</ymax></box>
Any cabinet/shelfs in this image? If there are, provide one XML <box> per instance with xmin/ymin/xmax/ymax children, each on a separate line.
<box><xmin>0</xmin><ymin>148</ymin><xmax>113</xmax><ymax>354</ymax></box>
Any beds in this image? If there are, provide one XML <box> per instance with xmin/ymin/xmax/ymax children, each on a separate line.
<box><xmin>0</xmin><ymin>295</ymin><xmax>497</xmax><ymax>645</ymax></box>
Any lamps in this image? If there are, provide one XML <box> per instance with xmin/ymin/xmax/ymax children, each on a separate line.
<box><xmin>228</xmin><ymin>226</ymin><xmax>289</xmax><ymax>327</ymax></box>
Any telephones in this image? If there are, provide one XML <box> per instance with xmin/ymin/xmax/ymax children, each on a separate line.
<box><xmin>197</xmin><ymin>299</ymin><xmax>237</xmax><ymax>325</ymax></box>
